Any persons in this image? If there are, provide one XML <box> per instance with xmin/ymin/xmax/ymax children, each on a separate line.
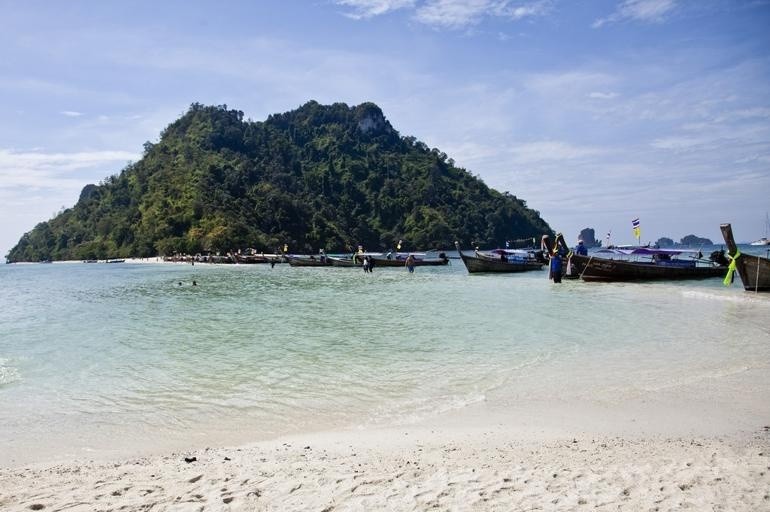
<box><xmin>320</xmin><ymin>247</ymin><xmax>323</xmax><ymax>255</ymax></box>
<box><xmin>500</xmin><ymin>240</ymin><xmax>588</xmax><ymax>283</ymax></box>
<box><xmin>358</xmin><ymin>240</ymin><xmax>414</xmax><ymax>273</ymax></box>
<box><xmin>284</xmin><ymin>244</ymin><xmax>288</xmax><ymax>254</ymax></box>
<box><xmin>156</xmin><ymin>248</ymin><xmax>275</xmax><ymax>268</ymax></box>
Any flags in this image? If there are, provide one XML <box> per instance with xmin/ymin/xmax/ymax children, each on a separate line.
<box><xmin>631</xmin><ymin>217</ymin><xmax>640</xmax><ymax>239</ymax></box>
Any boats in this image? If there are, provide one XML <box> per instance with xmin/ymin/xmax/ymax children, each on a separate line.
<box><xmin>554</xmin><ymin>233</ymin><xmax>733</xmax><ymax>281</ymax></box>
<box><xmin>454</xmin><ymin>240</ymin><xmax>544</xmax><ymax>272</ymax></box>
<box><xmin>749</xmin><ymin>235</ymin><xmax>770</xmax><ymax>246</ymax></box>
<box><xmin>353</xmin><ymin>245</ymin><xmax>449</xmax><ymax>269</ymax></box>
<box><xmin>84</xmin><ymin>259</ymin><xmax>97</xmax><ymax>263</ymax></box>
<box><xmin>318</xmin><ymin>248</ymin><xmax>362</xmax><ymax>269</ymax></box>
<box><xmin>106</xmin><ymin>258</ymin><xmax>126</xmax><ymax>264</ymax></box>
<box><xmin>540</xmin><ymin>233</ymin><xmax>580</xmax><ymax>279</ymax></box>
<box><xmin>718</xmin><ymin>221</ymin><xmax>770</xmax><ymax>293</ymax></box>
<box><xmin>163</xmin><ymin>250</ymin><xmax>286</xmax><ymax>265</ymax></box>
<box><xmin>284</xmin><ymin>252</ymin><xmax>329</xmax><ymax>268</ymax></box>
